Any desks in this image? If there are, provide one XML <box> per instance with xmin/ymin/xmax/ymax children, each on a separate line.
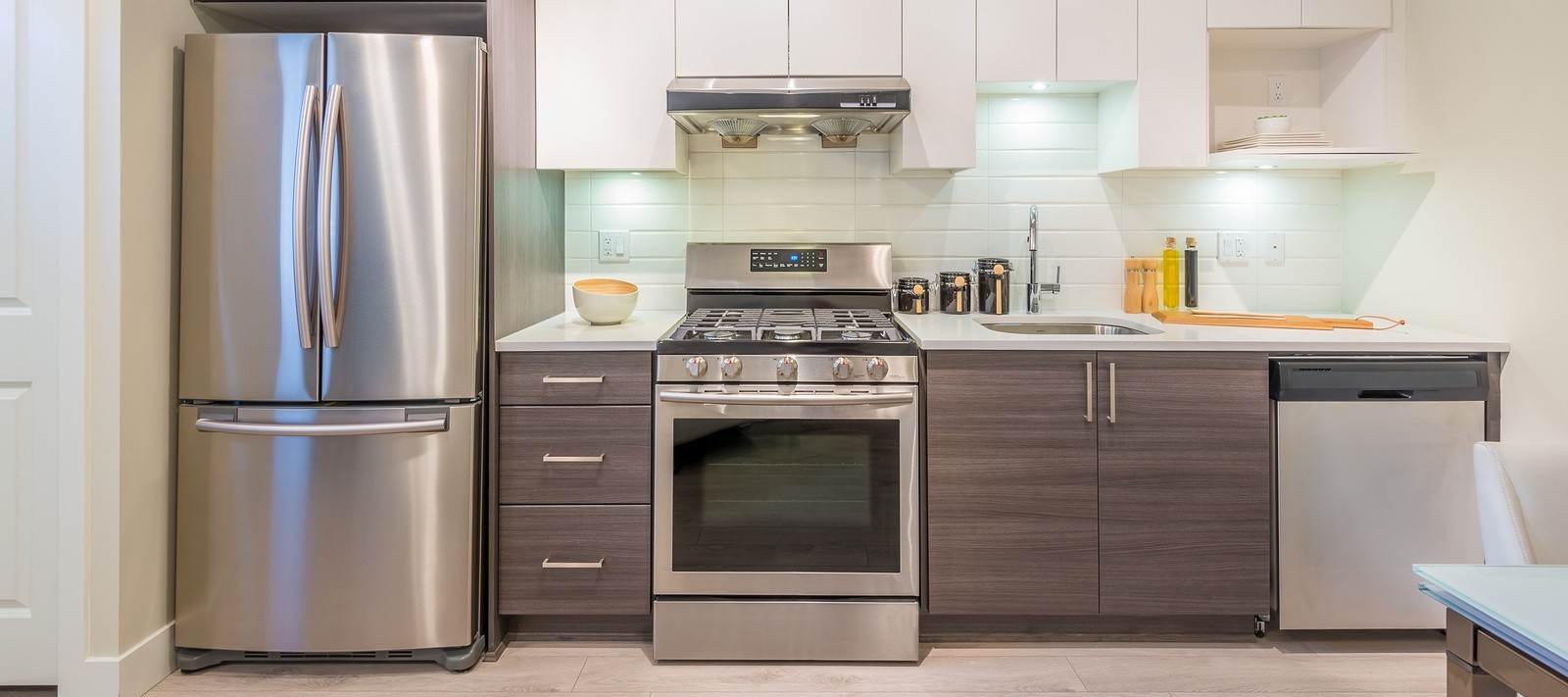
<box><xmin>1412</xmin><ymin>563</ymin><xmax>1568</xmax><ymax>697</ymax></box>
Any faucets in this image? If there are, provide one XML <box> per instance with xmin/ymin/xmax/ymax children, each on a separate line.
<box><xmin>1025</xmin><ymin>204</ymin><xmax>1062</xmax><ymax>314</ymax></box>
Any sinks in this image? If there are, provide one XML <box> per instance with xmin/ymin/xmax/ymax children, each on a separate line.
<box><xmin>971</xmin><ymin>314</ymin><xmax>1165</xmax><ymax>337</ymax></box>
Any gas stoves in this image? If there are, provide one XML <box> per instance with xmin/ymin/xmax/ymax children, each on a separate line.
<box><xmin>656</xmin><ymin>242</ymin><xmax>919</xmax><ymax>384</ymax></box>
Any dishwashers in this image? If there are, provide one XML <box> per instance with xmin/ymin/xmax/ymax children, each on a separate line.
<box><xmin>1269</xmin><ymin>360</ymin><xmax>1491</xmax><ymax>632</ymax></box>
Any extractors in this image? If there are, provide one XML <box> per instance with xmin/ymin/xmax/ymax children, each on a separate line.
<box><xmin>666</xmin><ymin>77</ymin><xmax>912</xmax><ymax>145</ymax></box>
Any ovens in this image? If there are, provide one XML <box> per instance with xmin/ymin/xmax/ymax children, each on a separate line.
<box><xmin>651</xmin><ymin>384</ymin><xmax>920</xmax><ymax>662</ymax></box>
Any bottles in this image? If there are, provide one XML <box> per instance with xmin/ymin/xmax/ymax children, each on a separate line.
<box><xmin>934</xmin><ymin>271</ymin><xmax>976</xmax><ymax>314</ymax></box>
<box><xmin>1184</xmin><ymin>236</ymin><xmax>1199</xmax><ymax>308</ymax></box>
<box><xmin>891</xmin><ymin>275</ymin><xmax>933</xmax><ymax>316</ymax></box>
<box><xmin>1141</xmin><ymin>257</ymin><xmax>1159</xmax><ymax>314</ymax></box>
<box><xmin>1123</xmin><ymin>258</ymin><xmax>1141</xmax><ymax>314</ymax></box>
<box><xmin>1161</xmin><ymin>237</ymin><xmax>1180</xmax><ymax>311</ymax></box>
<box><xmin>973</xmin><ymin>257</ymin><xmax>1015</xmax><ymax>316</ymax></box>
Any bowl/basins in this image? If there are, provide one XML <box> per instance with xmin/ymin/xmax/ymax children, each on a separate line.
<box><xmin>1253</xmin><ymin>116</ymin><xmax>1291</xmax><ymax>133</ymax></box>
<box><xmin>572</xmin><ymin>279</ymin><xmax>639</xmax><ymax>326</ymax></box>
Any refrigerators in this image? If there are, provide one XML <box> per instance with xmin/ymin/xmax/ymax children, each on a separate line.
<box><xmin>171</xmin><ymin>29</ymin><xmax>491</xmax><ymax>674</ymax></box>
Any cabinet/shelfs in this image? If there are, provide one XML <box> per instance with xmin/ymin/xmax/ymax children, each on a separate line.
<box><xmin>534</xmin><ymin>0</ymin><xmax>1419</xmax><ymax>175</ymax></box>
<box><xmin>498</xmin><ymin>351</ymin><xmax>652</xmax><ymax>641</ymax></box>
<box><xmin>919</xmin><ymin>350</ymin><xmax>1269</xmax><ymax>643</ymax></box>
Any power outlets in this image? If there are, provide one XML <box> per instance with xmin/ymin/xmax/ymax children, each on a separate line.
<box><xmin>1269</xmin><ymin>77</ymin><xmax>1287</xmax><ymax>106</ymax></box>
<box><xmin>1217</xmin><ymin>233</ymin><xmax>1249</xmax><ymax>267</ymax></box>
<box><xmin>599</xmin><ymin>231</ymin><xmax>630</xmax><ymax>262</ymax></box>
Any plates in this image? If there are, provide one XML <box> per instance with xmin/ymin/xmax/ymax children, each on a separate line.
<box><xmin>1213</xmin><ymin>132</ymin><xmax>1334</xmax><ymax>152</ymax></box>
